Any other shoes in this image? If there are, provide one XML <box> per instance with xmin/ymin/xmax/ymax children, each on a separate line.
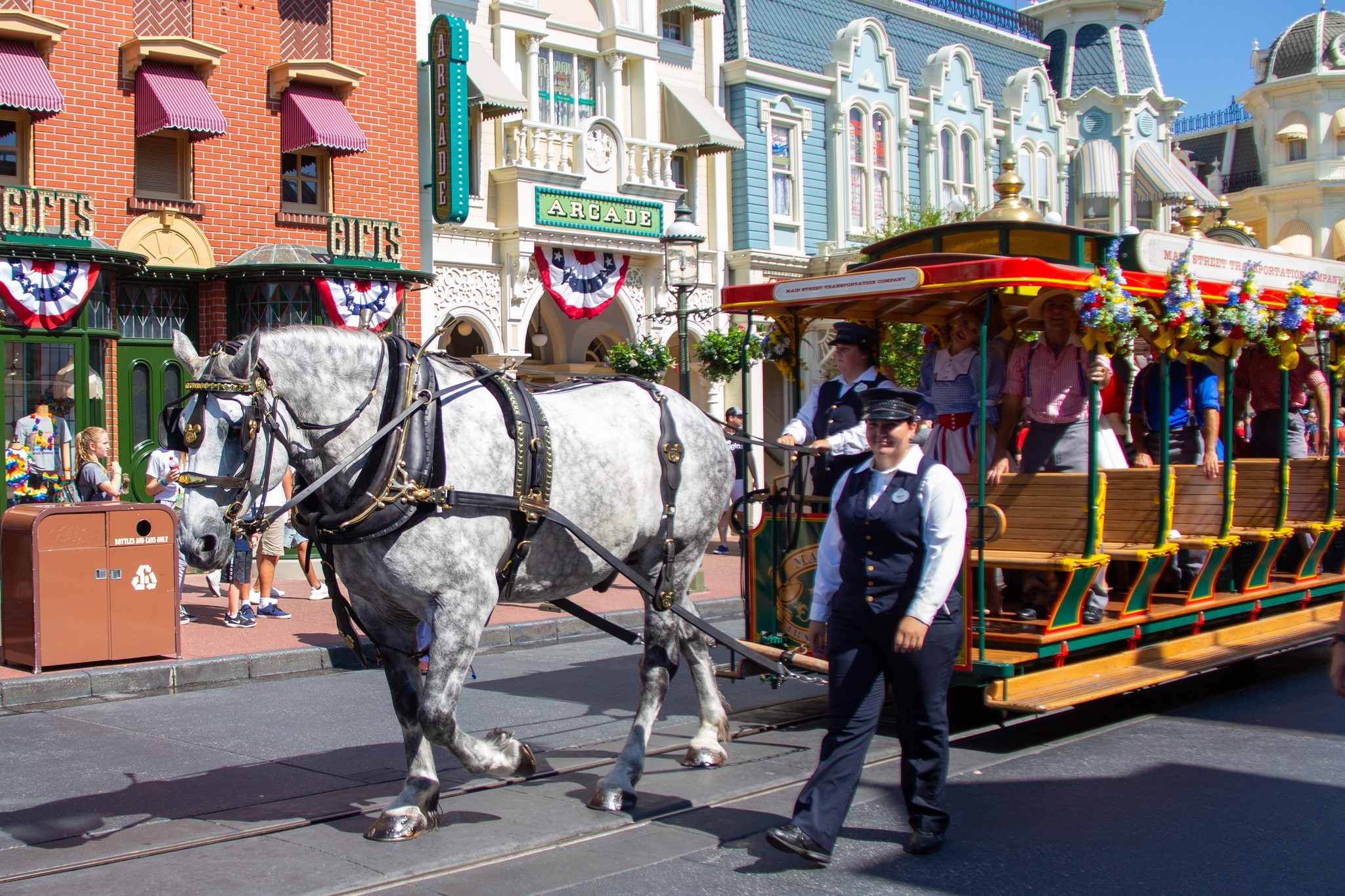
<box><xmin>270</xmin><ymin>588</ymin><xmax>285</xmax><ymax>597</ymax></box>
<box><xmin>713</xmin><ymin>545</ymin><xmax>730</xmax><ymax>554</ymax></box>
<box><xmin>419</xmin><ymin>661</ymin><xmax>429</xmax><ymax>675</ymax></box>
<box><xmin>974</xmin><ymin>599</ymin><xmax>1003</xmax><ymax>614</ymax></box>
<box><xmin>206</xmin><ymin>569</ymin><xmax>221</xmax><ymax>597</ymax></box>
<box><xmin>249</xmin><ymin>587</ymin><xmax>278</xmax><ymax>605</ymax></box>
<box><xmin>1153</xmin><ymin>582</ymin><xmax>1182</xmax><ymax>593</ymax></box>
<box><xmin>309</xmin><ymin>581</ymin><xmax>330</xmax><ymax>600</ymax></box>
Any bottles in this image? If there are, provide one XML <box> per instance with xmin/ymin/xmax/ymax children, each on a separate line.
<box><xmin>169</xmin><ymin>456</ymin><xmax>180</xmax><ymax>483</ymax></box>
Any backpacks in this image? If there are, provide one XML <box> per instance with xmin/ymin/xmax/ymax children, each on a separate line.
<box><xmin>54</xmin><ymin>461</ymin><xmax>105</xmax><ymax>503</ymax></box>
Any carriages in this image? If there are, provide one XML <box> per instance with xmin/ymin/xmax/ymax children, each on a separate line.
<box><xmin>151</xmin><ymin>214</ymin><xmax>1345</xmax><ymax>843</ymax></box>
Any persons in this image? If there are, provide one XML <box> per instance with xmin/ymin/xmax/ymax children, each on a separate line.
<box><xmin>13</xmin><ymin>405</ymin><xmax>73</xmax><ymax>504</ymax></box>
<box><xmin>985</xmin><ymin>286</ymin><xmax>1130</xmax><ymax>486</ymax></box>
<box><xmin>1130</xmin><ymin>361</ymin><xmax>1225</xmax><ymax>478</ymax></box>
<box><xmin>145</xmin><ymin>448</ymin><xmax>180</xmax><ymax>510</ymax></box>
<box><xmin>75</xmin><ymin>427</ymin><xmax>129</xmax><ymax>503</ymax></box>
<box><xmin>777</xmin><ymin>321</ymin><xmax>898</xmax><ymax>513</ymax></box>
<box><xmin>178</xmin><ymin>549</ymin><xmax>198</xmax><ymax>625</ymax></box>
<box><xmin>1223</xmin><ymin>322</ymin><xmax>1330</xmax><ymax>461</ymax></box>
<box><xmin>206</xmin><ymin>463</ymin><xmax>331</xmax><ymax>628</ymax></box>
<box><xmin>713</xmin><ymin>406</ymin><xmax>762</xmax><ymax>554</ymax></box>
<box><xmin>765</xmin><ymin>389</ymin><xmax>967</xmax><ymax>865</ymax></box>
<box><xmin>912</xmin><ymin>304</ymin><xmax>1017</xmax><ymax>482</ymax></box>
<box><xmin>1236</xmin><ymin>407</ymin><xmax>1345</xmax><ymax>455</ymax></box>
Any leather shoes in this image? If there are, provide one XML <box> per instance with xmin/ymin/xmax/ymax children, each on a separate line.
<box><xmin>1016</xmin><ymin>602</ymin><xmax>1049</xmax><ymax>620</ymax></box>
<box><xmin>1082</xmin><ymin>605</ymin><xmax>1104</xmax><ymax>624</ymax></box>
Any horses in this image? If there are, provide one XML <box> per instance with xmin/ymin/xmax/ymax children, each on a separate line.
<box><xmin>162</xmin><ymin>320</ymin><xmax>740</xmax><ymax>847</ymax></box>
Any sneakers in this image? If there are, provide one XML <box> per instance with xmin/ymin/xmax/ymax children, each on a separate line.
<box><xmin>224</xmin><ymin>611</ymin><xmax>252</xmax><ymax>624</ymax></box>
<box><xmin>180</xmin><ymin>605</ymin><xmax>198</xmax><ymax>622</ymax></box>
<box><xmin>227</xmin><ymin>611</ymin><xmax>257</xmax><ymax>627</ymax></box>
<box><xmin>256</xmin><ymin>602</ymin><xmax>292</xmax><ymax>618</ymax></box>
<box><xmin>909</xmin><ymin>832</ymin><xmax>943</xmax><ymax>855</ymax></box>
<box><xmin>765</xmin><ymin>824</ymin><xmax>833</xmax><ymax>863</ymax></box>
<box><xmin>180</xmin><ymin>613</ymin><xmax>190</xmax><ymax>624</ymax></box>
<box><xmin>240</xmin><ymin>604</ymin><xmax>255</xmax><ymax>622</ymax></box>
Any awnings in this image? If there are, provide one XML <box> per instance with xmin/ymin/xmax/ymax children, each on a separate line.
<box><xmin>1134</xmin><ymin>141</ymin><xmax>1190</xmax><ymax>202</ymax></box>
<box><xmin>134</xmin><ymin>59</ymin><xmax>227</xmax><ymax>143</ymax></box>
<box><xmin>659</xmin><ymin>79</ymin><xmax>746</xmax><ymax>158</ymax></box>
<box><xmin>0</xmin><ymin>35</ymin><xmax>64</xmax><ymax>124</ymax></box>
<box><xmin>1171</xmin><ymin>151</ymin><xmax>1221</xmax><ymax>212</ymax></box>
<box><xmin>1075</xmin><ymin>138</ymin><xmax>1119</xmax><ymax>202</ymax></box>
<box><xmin>281</xmin><ymin>80</ymin><xmax>367</xmax><ymax>159</ymax></box>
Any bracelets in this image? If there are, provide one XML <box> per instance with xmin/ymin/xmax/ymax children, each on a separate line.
<box><xmin>63</xmin><ymin>467</ymin><xmax>71</xmax><ymax>471</ymax></box>
<box><xmin>161</xmin><ymin>479</ymin><xmax>168</xmax><ymax>487</ymax></box>
<box><xmin>113</xmin><ymin>473</ymin><xmax>122</xmax><ymax>476</ymax></box>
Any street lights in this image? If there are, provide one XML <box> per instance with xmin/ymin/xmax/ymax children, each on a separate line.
<box><xmin>658</xmin><ymin>193</ymin><xmax>707</xmax><ymax>405</ymax></box>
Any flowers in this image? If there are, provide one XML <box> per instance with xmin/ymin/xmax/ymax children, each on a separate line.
<box><xmin>1159</xmin><ymin>236</ymin><xmax>1210</xmax><ymax>352</ymax></box>
<box><xmin>603</xmin><ymin>332</ymin><xmax>682</xmax><ymax>386</ymax></box>
<box><xmin>1271</xmin><ymin>270</ymin><xmax>1328</xmax><ymax>342</ymax></box>
<box><xmin>1072</xmin><ymin>228</ymin><xmax>1162</xmax><ymax>361</ymax></box>
<box><xmin>760</xmin><ymin>320</ymin><xmax>809</xmax><ymax>384</ymax></box>
<box><xmin>692</xmin><ymin>320</ymin><xmax>768</xmax><ymax>384</ymax></box>
<box><xmin>1324</xmin><ymin>274</ymin><xmax>1345</xmax><ymax>335</ymax></box>
<box><xmin>1205</xmin><ymin>257</ymin><xmax>1283</xmax><ymax>369</ymax></box>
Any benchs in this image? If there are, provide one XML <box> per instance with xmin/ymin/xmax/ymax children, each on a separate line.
<box><xmin>1308</xmin><ymin>453</ymin><xmax>1345</xmax><ymax>576</ymax></box>
<box><xmin>952</xmin><ymin>471</ymin><xmax>1111</xmax><ymax>638</ymax></box>
<box><xmin>1237</xmin><ymin>455</ymin><xmax>1344</xmax><ymax>584</ymax></box>
<box><xmin>1095</xmin><ymin>465</ymin><xmax>1180</xmax><ymax>622</ymax></box>
<box><xmin>1218</xmin><ymin>460</ymin><xmax>1295</xmax><ymax>595</ymax></box>
<box><xmin>1133</xmin><ymin>464</ymin><xmax>1241</xmax><ymax>606</ymax></box>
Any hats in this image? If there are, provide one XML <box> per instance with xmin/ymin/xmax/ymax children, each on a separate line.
<box><xmin>1139</xmin><ymin>317</ymin><xmax>1197</xmax><ymax>351</ymax></box>
<box><xmin>828</xmin><ymin>322</ymin><xmax>875</xmax><ymax>346</ymax></box>
<box><xmin>858</xmin><ymin>388</ymin><xmax>926</xmax><ymax>420</ymax></box>
<box><xmin>725</xmin><ymin>407</ymin><xmax>748</xmax><ymax>416</ymax></box>
<box><xmin>1302</xmin><ymin>409</ymin><xmax>1310</xmax><ymax>414</ymax></box>
<box><xmin>1027</xmin><ymin>286</ymin><xmax>1082</xmax><ymax>321</ymax></box>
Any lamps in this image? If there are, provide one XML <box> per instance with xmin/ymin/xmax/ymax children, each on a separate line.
<box><xmin>531</xmin><ymin>299</ymin><xmax>549</xmax><ymax>347</ymax></box>
<box><xmin>458</xmin><ymin>321</ymin><xmax>473</xmax><ymax>337</ymax></box>
